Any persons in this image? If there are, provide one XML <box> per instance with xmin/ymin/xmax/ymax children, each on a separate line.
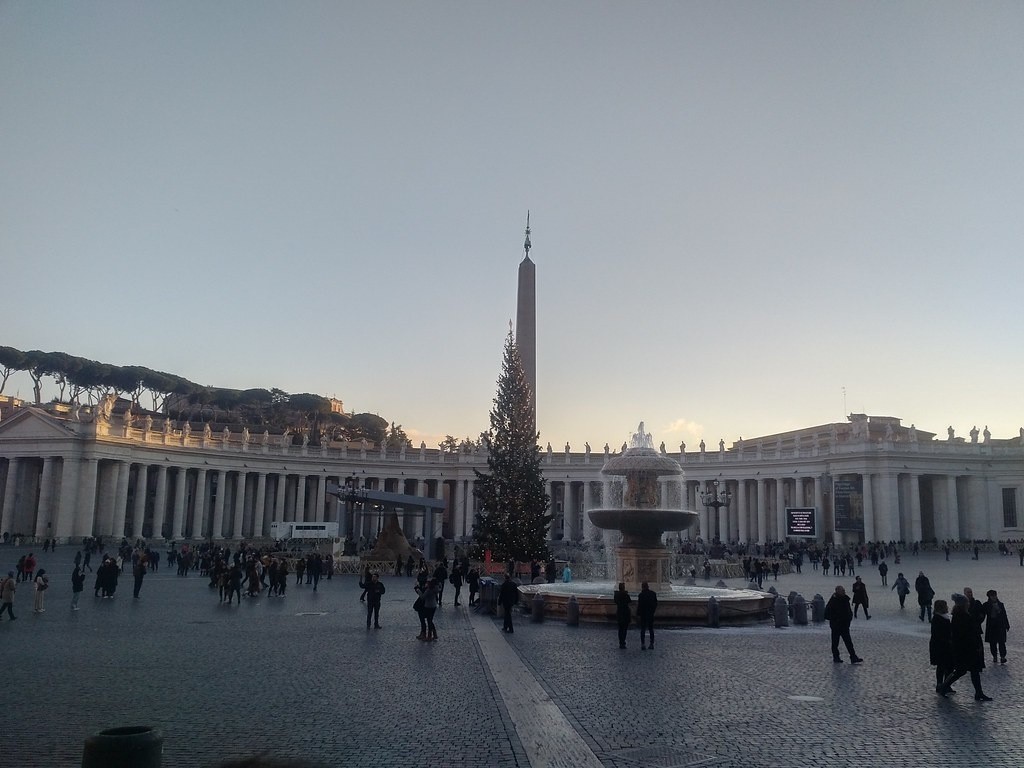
<box><xmin>547</xmin><ymin>441</ymin><xmax>627</xmax><ymax>456</ymax></box>
<box><xmin>892</xmin><ymin>573</ymin><xmax>910</xmax><ymax>609</ymax></box>
<box><xmin>125</xmin><ymin>409</ymin><xmax>308</xmax><ymax>449</ymax></box>
<box><xmin>660</xmin><ymin>421</ymin><xmax>868</xmax><ymax>456</ymax></box>
<box><xmin>666</xmin><ymin>536</ymin><xmax>1024</xmax><ymax>589</ymax></box>
<box><xmin>614</xmin><ymin>582</ymin><xmax>658</xmax><ymax>650</ymax></box>
<box><xmin>394</xmin><ymin>552</ymin><xmax>571</xmax><ymax>642</ymax></box>
<box><xmin>70</xmin><ymin>401</ymin><xmax>82</xmax><ymax>418</ymax></box>
<box><xmin>852</xmin><ymin>576</ymin><xmax>871</xmax><ymax>619</ymax></box>
<box><xmin>886</xmin><ymin>422</ymin><xmax>991</xmax><ymax>443</ymax></box>
<box><xmin>346</xmin><ymin>536</ymin><xmax>425</xmax><ymax>555</ymax></box>
<box><xmin>915</xmin><ymin>571</ymin><xmax>934</xmax><ymax>623</ymax></box>
<box><xmin>929</xmin><ymin>587</ymin><xmax>1010</xmax><ymax>701</ymax></box>
<box><xmin>320</xmin><ymin>435</ymin><xmax>475</xmax><ymax>454</ymax></box>
<box><xmin>824</xmin><ymin>585</ymin><xmax>863</xmax><ymax>663</ymax></box>
<box><xmin>0</xmin><ymin>532</ymin><xmax>334</xmax><ymax>620</ymax></box>
<box><xmin>359</xmin><ymin>567</ymin><xmax>386</xmax><ymax>629</ymax></box>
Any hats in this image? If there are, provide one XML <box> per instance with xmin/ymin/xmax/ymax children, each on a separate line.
<box><xmin>951</xmin><ymin>593</ymin><xmax>969</xmax><ymax>606</ymax></box>
<box><xmin>8</xmin><ymin>570</ymin><xmax>14</xmax><ymax>575</ymax></box>
<box><xmin>105</xmin><ymin>559</ymin><xmax>110</xmax><ymax>563</ymax></box>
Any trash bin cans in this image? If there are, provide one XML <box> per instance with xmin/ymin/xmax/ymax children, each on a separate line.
<box><xmin>479</xmin><ymin>577</ymin><xmax>507</xmax><ymax>617</ymax></box>
<box><xmin>82</xmin><ymin>725</ymin><xmax>164</xmax><ymax>768</ymax></box>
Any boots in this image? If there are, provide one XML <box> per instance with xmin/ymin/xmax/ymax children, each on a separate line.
<box><xmin>415</xmin><ymin>626</ymin><xmax>438</xmax><ymax>640</ymax></box>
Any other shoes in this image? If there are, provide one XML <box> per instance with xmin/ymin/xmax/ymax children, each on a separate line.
<box><xmin>919</xmin><ymin>615</ymin><xmax>924</xmax><ymax>621</ymax></box>
<box><xmin>939</xmin><ymin>683</ymin><xmax>956</xmax><ymax>695</ymax></box>
<box><xmin>9</xmin><ymin>594</ymin><xmax>114</xmax><ymax>622</ymax></box>
<box><xmin>619</xmin><ymin>641</ymin><xmax>626</xmax><ymax>649</ymax></box>
<box><xmin>374</xmin><ymin>624</ymin><xmax>382</xmax><ymax>629</ymax></box>
<box><xmin>975</xmin><ymin>693</ymin><xmax>992</xmax><ymax>701</ymax></box>
<box><xmin>642</xmin><ymin>646</ymin><xmax>646</xmax><ymax>650</ymax></box>
<box><xmin>994</xmin><ymin>657</ymin><xmax>997</xmax><ymax>662</ymax></box>
<box><xmin>833</xmin><ymin>655</ymin><xmax>863</xmax><ymax>664</ymax></box>
<box><xmin>866</xmin><ymin>616</ymin><xmax>871</xmax><ymax>620</ymax></box>
<box><xmin>648</xmin><ymin>644</ymin><xmax>654</xmax><ymax>649</ymax></box>
<box><xmin>502</xmin><ymin>628</ymin><xmax>514</xmax><ymax>633</ymax></box>
<box><xmin>453</xmin><ymin>602</ymin><xmax>461</xmax><ymax>606</ymax></box>
<box><xmin>1000</xmin><ymin>657</ymin><xmax>1006</xmax><ymax>663</ymax></box>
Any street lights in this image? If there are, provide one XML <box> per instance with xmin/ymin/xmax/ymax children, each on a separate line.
<box><xmin>336</xmin><ymin>472</ymin><xmax>370</xmax><ymax>541</ymax></box>
<box><xmin>700</xmin><ymin>479</ymin><xmax>733</xmax><ymax>543</ymax></box>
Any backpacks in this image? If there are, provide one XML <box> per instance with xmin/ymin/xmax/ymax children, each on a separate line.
<box><xmin>26</xmin><ymin>558</ymin><xmax>33</xmax><ymax>568</ymax></box>
<box><xmin>449</xmin><ymin>574</ymin><xmax>457</xmax><ymax>584</ymax></box>
<box><xmin>824</xmin><ymin>593</ymin><xmax>837</xmax><ymax>620</ymax></box>
<box><xmin>466</xmin><ymin>574</ymin><xmax>471</xmax><ymax>583</ymax></box>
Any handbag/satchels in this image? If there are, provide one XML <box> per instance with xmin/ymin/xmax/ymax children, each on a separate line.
<box><xmin>38</xmin><ymin>584</ymin><xmax>48</xmax><ymax>591</ymax></box>
<box><xmin>904</xmin><ymin>587</ymin><xmax>909</xmax><ymax>594</ymax></box>
